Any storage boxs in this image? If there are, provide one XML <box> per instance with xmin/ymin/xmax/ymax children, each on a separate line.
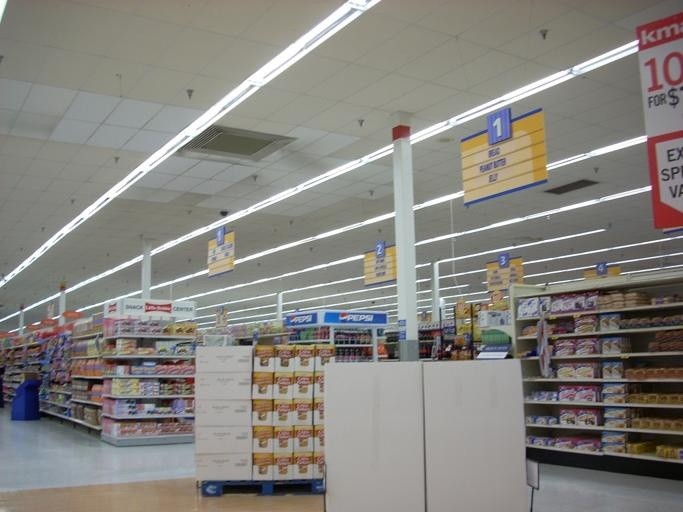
<box><xmin>196</xmin><ymin>342</ymin><xmax>335</xmax><ymax>481</ymax></box>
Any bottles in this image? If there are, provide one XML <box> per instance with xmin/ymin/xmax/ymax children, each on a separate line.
<box><xmin>286</xmin><ymin>319</ymin><xmax>455</xmax><ymax>360</ymax></box>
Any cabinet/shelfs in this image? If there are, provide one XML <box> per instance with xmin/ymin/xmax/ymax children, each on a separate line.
<box><xmin>323</xmin><ymin>355</ymin><xmax>530</xmax><ymax>512</ymax></box>
<box><xmin>0</xmin><ymin>299</ymin><xmax>197</xmax><ymax>449</ymax></box>
<box><xmin>501</xmin><ymin>271</ymin><xmax>682</xmax><ymax>481</ymax></box>
